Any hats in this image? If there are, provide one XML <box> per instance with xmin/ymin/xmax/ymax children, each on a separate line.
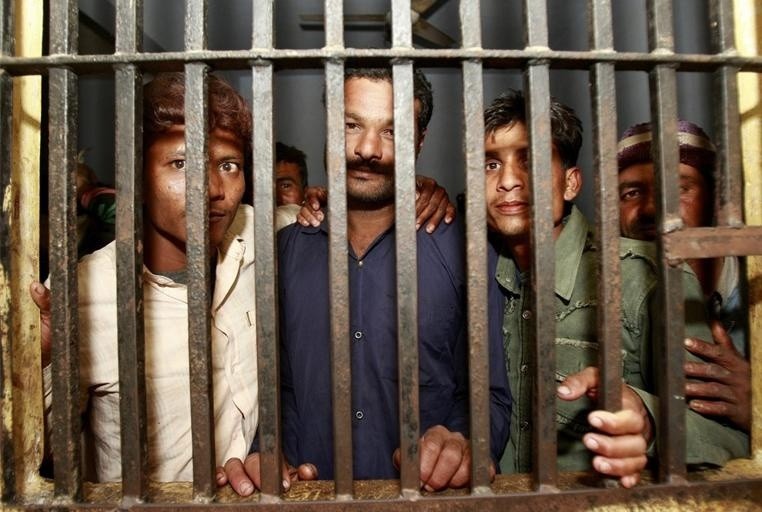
<box><xmin>619</xmin><ymin>118</ymin><xmax>716</xmax><ymax>173</ymax></box>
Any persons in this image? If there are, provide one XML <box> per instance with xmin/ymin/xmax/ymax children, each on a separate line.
<box><xmin>31</xmin><ymin>73</ymin><xmax>455</xmax><ymax>477</ymax></box>
<box><xmin>617</xmin><ymin>120</ymin><xmax>753</xmax><ymax>430</ymax></box>
<box><xmin>215</xmin><ymin>60</ymin><xmax>503</xmax><ymax>496</ymax></box>
<box><xmin>273</xmin><ymin>141</ymin><xmax>309</xmax><ymax>207</ymax></box>
<box><xmin>297</xmin><ymin>90</ymin><xmax>750</xmax><ymax>490</ymax></box>
